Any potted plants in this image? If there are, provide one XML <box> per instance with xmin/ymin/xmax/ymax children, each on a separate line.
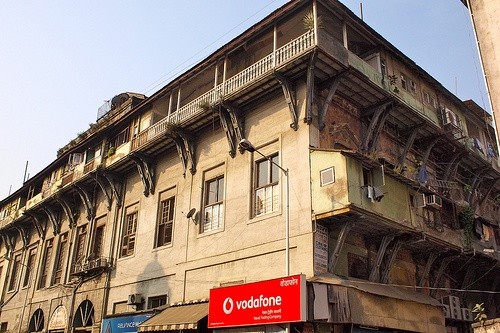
<box><xmin>107</xmin><ymin>146</ymin><xmax>116</xmax><ymax>158</ymax></box>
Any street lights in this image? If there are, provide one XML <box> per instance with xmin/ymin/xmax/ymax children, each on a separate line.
<box><xmin>239</xmin><ymin>139</ymin><xmax>290</xmax><ymax>277</ymax></box>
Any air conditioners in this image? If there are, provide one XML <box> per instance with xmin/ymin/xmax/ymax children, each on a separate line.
<box><xmin>127</xmin><ymin>293</ymin><xmax>142</xmax><ymax>306</ymax></box>
<box><xmin>95</xmin><ymin>258</ymin><xmax>107</xmax><ymax>268</ymax></box>
<box><xmin>461</xmin><ymin>308</ymin><xmax>474</xmax><ymax>322</ymax></box>
<box><xmin>442</xmin><ymin>304</ymin><xmax>451</xmax><ymax>320</ymax></box>
<box><xmin>425</xmin><ymin>195</ymin><xmax>443</xmax><ymax>210</ymax></box>
<box><xmin>449</xmin><ymin>295</ymin><xmax>463</xmax><ymax>320</ymax></box>
<box><xmin>440</xmin><ymin>108</ymin><xmax>458</xmax><ymax>130</ymax></box>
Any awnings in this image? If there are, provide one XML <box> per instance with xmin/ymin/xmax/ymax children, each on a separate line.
<box><xmin>136</xmin><ymin>297</ymin><xmax>209</xmax><ymax>332</ymax></box>
